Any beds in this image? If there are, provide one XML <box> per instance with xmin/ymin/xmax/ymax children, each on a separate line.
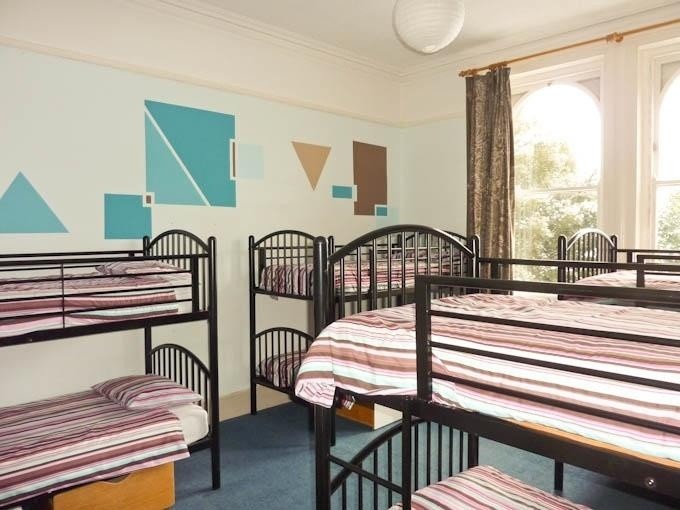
<box><xmin>251</xmin><ymin>224</ymin><xmax>679</xmax><ymax>506</ymax></box>
<box><xmin>1</xmin><ymin>229</ymin><xmax>220</xmax><ymax>506</ymax></box>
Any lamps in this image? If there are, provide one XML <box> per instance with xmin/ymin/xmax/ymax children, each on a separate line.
<box><xmin>391</xmin><ymin>0</ymin><xmax>468</xmax><ymax>55</ymax></box>
